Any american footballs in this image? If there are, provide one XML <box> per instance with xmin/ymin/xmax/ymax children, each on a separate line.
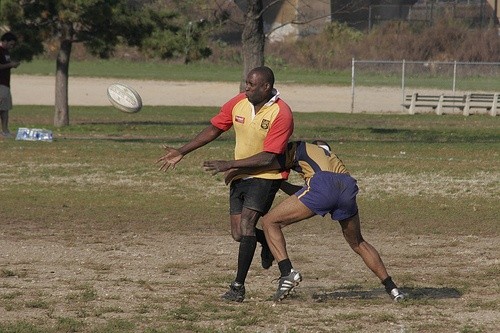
<box><xmin>105</xmin><ymin>82</ymin><xmax>142</xmax><ymax>113</ymax></box>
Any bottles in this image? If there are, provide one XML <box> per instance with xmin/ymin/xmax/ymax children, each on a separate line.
<box><xmin>16</xmin><ymin>128</ymin><xmax>52</xmax><ymax>141</ymax></box>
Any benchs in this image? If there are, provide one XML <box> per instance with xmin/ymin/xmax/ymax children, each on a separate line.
<box><xmin>402</xmin><ymin>92</ymin><xmax>500</xmax><ymax>117</ymax></box>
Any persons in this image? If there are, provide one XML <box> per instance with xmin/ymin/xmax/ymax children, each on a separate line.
<box><xmin>0</xmin><ymin>32</ymin><xmax>20</xmax><ymax>138</ymax></box>
<box><xmin>225</xmin><ymin>139</ymin><xmax>406</xmax><ymax>305</ymax></box>
<box><xmin>155</xmin><ymin>66</ymin><xmax>294</xmax><ymax>304</ymax></box>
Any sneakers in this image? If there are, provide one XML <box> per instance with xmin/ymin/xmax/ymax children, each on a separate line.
<box><xmin>271</xmin><ymin>270</ymin><xmax>302</xmax><ymax>302</ymax></box>
<box><xmin>220</xmin><ymin>284</ymin><xmax>246</xmax><ymax>302</ymax></box>
<box><xmin>388</xmin><ymin>287</ymin><xmax>404</xmax><ymax>301</ymax></box>
<box><xmin>261</xmin><ymin>245</ymin><xmax>276</xmax><ymax>269</ymax></box>
